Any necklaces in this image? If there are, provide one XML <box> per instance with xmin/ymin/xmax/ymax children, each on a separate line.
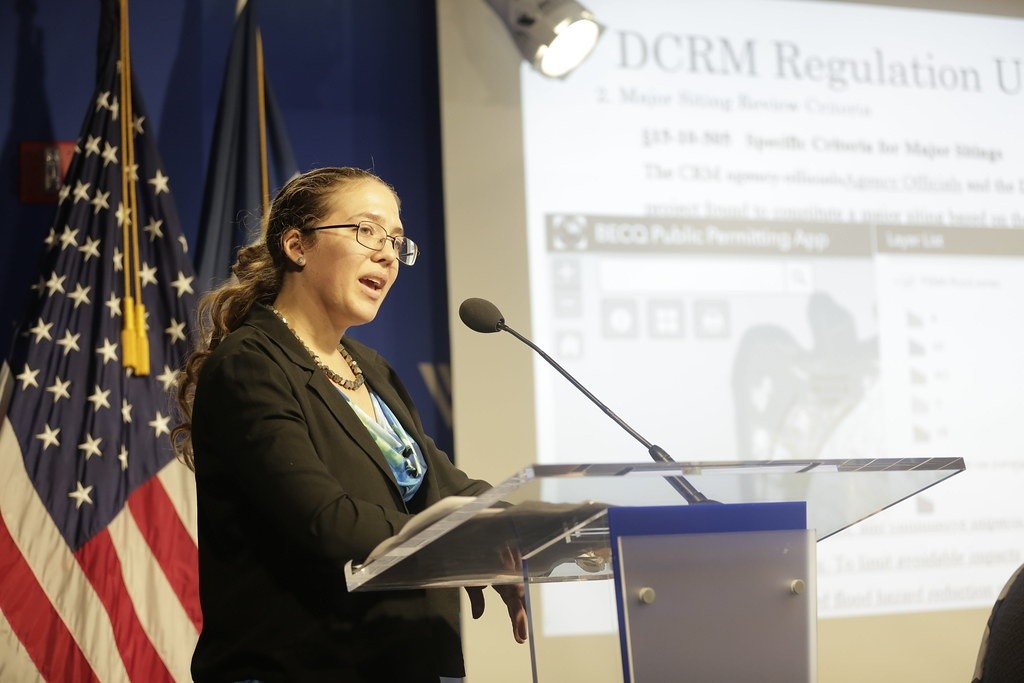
<box><xmin>267</xmin><ymin>303</ymin><xmax>365</xmax><ymax>390</ymax></box>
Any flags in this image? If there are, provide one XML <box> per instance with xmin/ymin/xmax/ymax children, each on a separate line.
<box><xmin>2</xmin><ymin>0</ymin><xmax>213</xmax><ymax>683</ymax></box>
<box><xmin>195</xmin><ymin>0</ymin><xmax>301</xmax><ymax>308</ymax></box>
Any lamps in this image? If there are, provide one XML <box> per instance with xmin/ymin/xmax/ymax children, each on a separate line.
<box><xmin>486</xmin><ymin>0</ymin><xmax>601</xmax><ymax>78</ymax></box>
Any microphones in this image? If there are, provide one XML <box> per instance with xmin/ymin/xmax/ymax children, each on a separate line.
<box><xmin>459</xmin><ymin>298</ymin><xmax>728</xmax><ymax>507</ymax></box>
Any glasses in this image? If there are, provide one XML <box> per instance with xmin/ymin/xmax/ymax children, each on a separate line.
<box><xmin>305</xmin><ymin>220</ymin><xmax>420</xmax><ymax>266</ymax></box>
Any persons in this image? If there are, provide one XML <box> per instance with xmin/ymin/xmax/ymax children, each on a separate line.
<box><xmin>171</xmin><ymin>167</ymin><xmax>530</xmax><ymax>683</ymax></box>
